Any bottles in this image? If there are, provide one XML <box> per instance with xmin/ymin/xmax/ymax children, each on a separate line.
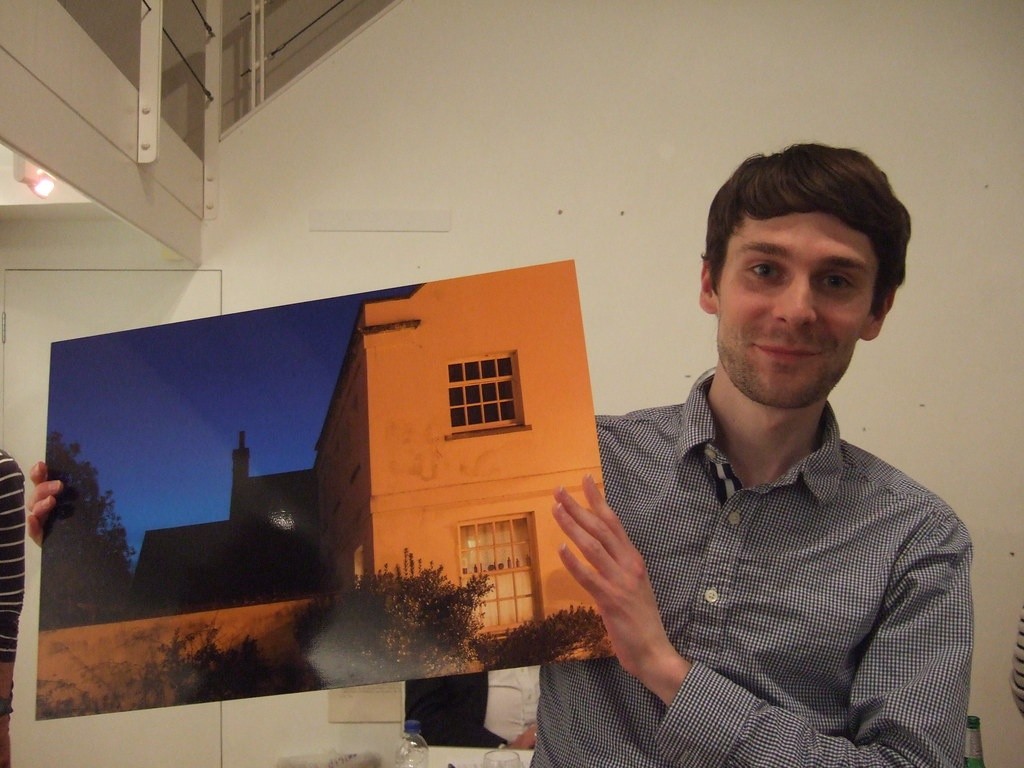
<box><xmin>395</xmin><ymin>720</ymin><xmax>429</xmax><ymax>768</ymax></box>
<box><xmin>965</xmin><ymin>716</ymin><xmax>986</xmax><ymax>768</ymax></box>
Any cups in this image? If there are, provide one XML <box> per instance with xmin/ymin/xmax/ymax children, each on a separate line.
<box><xmin>483</xmin><ymin>751</ymin><xmax>520</xmax><ymax>768</ymax></box>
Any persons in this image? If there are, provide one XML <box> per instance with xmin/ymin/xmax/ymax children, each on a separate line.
<box><xmin>28</xmin><ymin>142</ymin><xmax>974</xmax><ymax>768</ymax></box>
<box><xmin>0</xmin><ymin>447</ymin><xmax>25</xmax><ymax>768</ymax></box>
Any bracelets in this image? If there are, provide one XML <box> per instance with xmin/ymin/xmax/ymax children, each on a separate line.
<box><xmin>0</xmin><ymin>692</ymin><xmax>14</xmax><ymax>717</ymax></box>
